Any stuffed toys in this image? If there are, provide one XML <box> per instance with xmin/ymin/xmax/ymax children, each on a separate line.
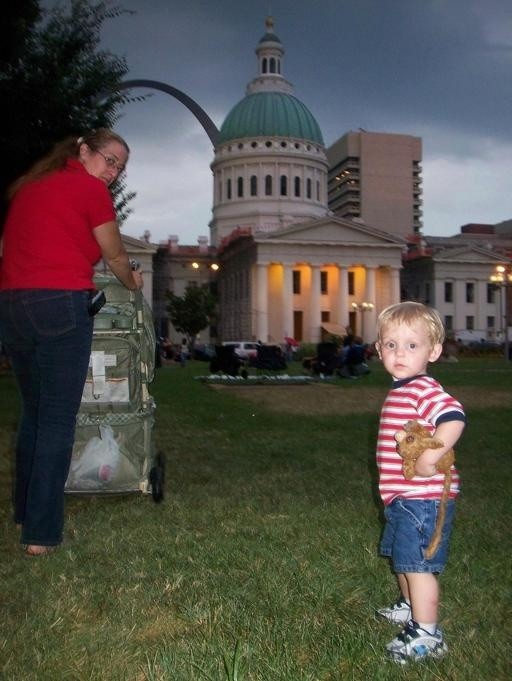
<box><xmin>392</xmin><ymin>418</ymin><xmax>458</xmax><ymax>560</ymax></box>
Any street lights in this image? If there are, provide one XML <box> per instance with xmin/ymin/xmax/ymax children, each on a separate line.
<box><xmin>490</xmin><ymin>265</ymin><xmax>512</xmax><ymax>359</ymax></box>
<box><xmin>351</xmin><ymin>302</ymin><xmax>373</xmax><ymax>339</ymax></box>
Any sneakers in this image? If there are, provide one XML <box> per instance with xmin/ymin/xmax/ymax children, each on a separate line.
<box><xmin>374</xmin><ymin>601</ymin><xmax>412</xmax><ymax>624</ymax></box>
<box><xmin>383</xmin><ymin>622</ymin><xmax>449</xmax><ymax>668</ymax></box>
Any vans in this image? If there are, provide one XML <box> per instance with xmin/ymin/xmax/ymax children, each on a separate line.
<box><xmin>454</xmin><ymin>330</ymin><xmax>488</xmax><ymax>345</ymax></box>
<box><xmin>222</xmin><ymin>341</ymin><xmax>261</xmax><ymax>359</ymax></box>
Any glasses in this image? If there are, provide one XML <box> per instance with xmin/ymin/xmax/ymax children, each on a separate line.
<box><xmin>98</xmin><ymin>150</ymin><xmax>123</xmax><ymax>173</ymax></box>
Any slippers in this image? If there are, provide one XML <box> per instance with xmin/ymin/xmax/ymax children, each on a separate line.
<box><xmin>15</xmin><ymin>515</ymin><xmax>54</xmax><ymax>558</ymax></box>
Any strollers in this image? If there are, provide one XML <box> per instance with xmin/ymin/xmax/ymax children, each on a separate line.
<box><xmin>62</xmin><ymin>262</ymin><xmax>167</xmax><ymax>502</ymax></box>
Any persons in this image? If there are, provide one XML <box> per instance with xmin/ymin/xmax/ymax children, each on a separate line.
<box><xmin>374</xmin><ymin>299</ymin><xmax>467</xmax><ymax>665</ymax></box>
<box><xmin>152</xmin><ymin>331</ymin><xmax>191</xmax><ymax>369</ymax></box>
<box><xmin>4</xmin><ymin>128</ymin><xmax>145</xmax><ymax>556</ymax></box>
<box><xmin>279</xmin><ymin>326</ymin><xmax>377</xmax><ymax>381</ymax></box>
